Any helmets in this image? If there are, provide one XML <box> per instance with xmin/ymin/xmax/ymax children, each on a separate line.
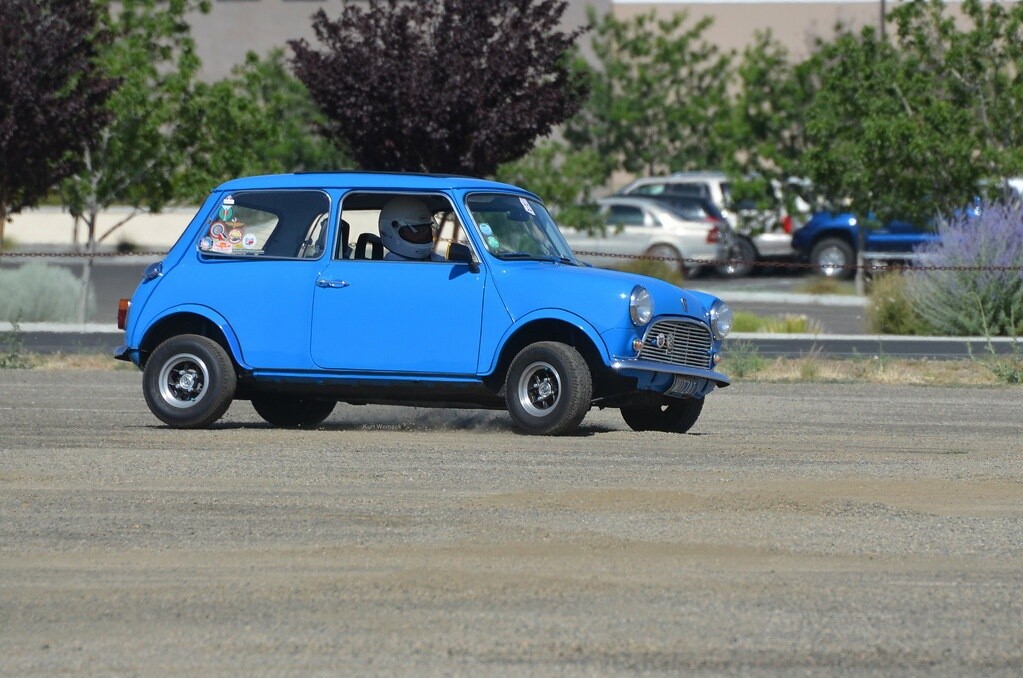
<box><xmin>378</xmin><ymin>197</ymin><xmax>434</xmax><ymax>258</ymax></box>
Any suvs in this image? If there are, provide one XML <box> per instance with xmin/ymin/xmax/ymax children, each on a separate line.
<box><xmin>600</xmin><ymin>171</ymin><xmax>810</xmax><ymax>281</ymax></box>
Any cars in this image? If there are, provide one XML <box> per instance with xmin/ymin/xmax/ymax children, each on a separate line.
<box><xmin>115</xmin><ymin>171</ymin><xmax>732</xmax><ymax>439</ymax></box>
<box><xmin>510</xmin><ymin>196</ymin><xmax>728</xmax><ymax>283</ymax></box>
<box><xmin>790</xmin><ymin>190</ymin><xmax>988</xmax><ymax>282</ymax></box>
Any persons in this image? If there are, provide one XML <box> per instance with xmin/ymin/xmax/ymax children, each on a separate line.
<box><xmin>378</xmin><ymin>195</ymin><xmax>449</xmax><ymax>261</ymax></box>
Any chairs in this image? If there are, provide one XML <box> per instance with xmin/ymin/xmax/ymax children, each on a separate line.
<box><xmin>313</xmin><ymin>218</ymin><xmax>349</xmax><ymax>260</ymax></box>
<box><xmin>354</xmin><ymin>233</ymin><xmax>384</xmax><ymax>260</ymax></box>
